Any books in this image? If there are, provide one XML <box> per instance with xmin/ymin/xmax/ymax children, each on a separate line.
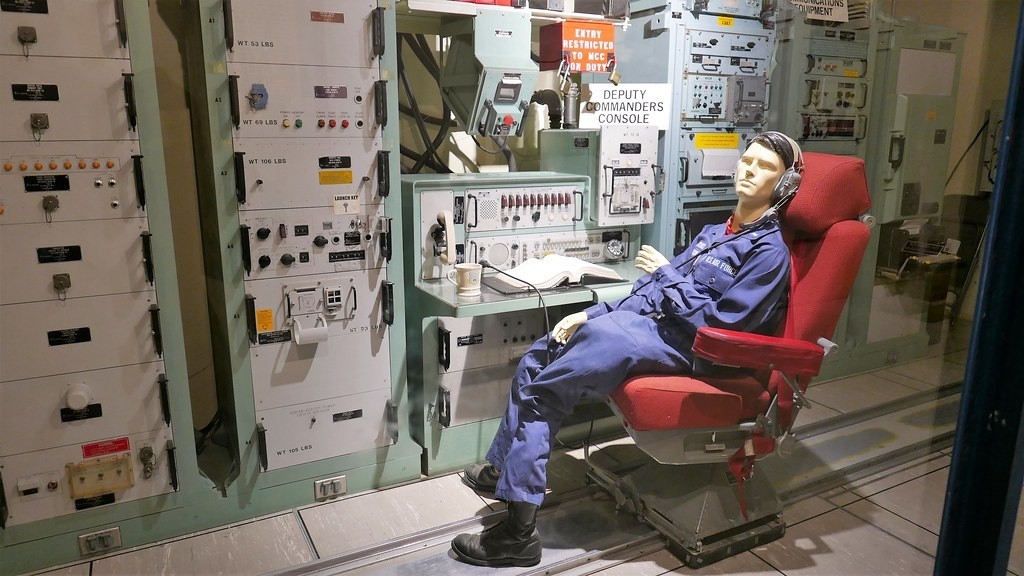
<box><xmin>482</xmin><ymin>254</ymin><xmax>628</xmax><ymax>294</ymax></box>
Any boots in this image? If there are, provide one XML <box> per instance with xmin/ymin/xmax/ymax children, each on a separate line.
<box><xmin>464</xmin><ymin>463</ymin><xmax>501</xmax><ymax>491</ymax></box>
<box><xmin>451</xmin><ymin>500</ymin><xmax>542</xmax><ymax>566</ymax></box>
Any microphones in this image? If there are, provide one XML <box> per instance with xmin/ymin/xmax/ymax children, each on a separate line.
<box><xmin>739</xmin><ymin>186</ymin><xmax>799</xmax><ymax>230</ymax></box>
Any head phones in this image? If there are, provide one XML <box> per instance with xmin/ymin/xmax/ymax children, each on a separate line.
<box><xmin>734</xmin><ymin>130</ymin><xmax>805</xmax><ymax>205</ymax></box>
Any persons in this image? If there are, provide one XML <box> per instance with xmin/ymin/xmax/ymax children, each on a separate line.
<box><xmin>451</xmin><ymin>132</ymin><xmax>803</xmax><ymax>567</ymax></box>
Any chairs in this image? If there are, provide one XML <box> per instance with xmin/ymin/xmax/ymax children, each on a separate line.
<box><xmin>570</xmin><ymin>152</ymin><xmax>876</xmax><ymax>568</ymax></box>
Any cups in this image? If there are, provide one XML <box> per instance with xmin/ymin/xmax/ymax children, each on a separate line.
<box><xmin>447</xmin><ymin>263</ymin><xmax>482</xmax><ymax>297</ymax></box>
